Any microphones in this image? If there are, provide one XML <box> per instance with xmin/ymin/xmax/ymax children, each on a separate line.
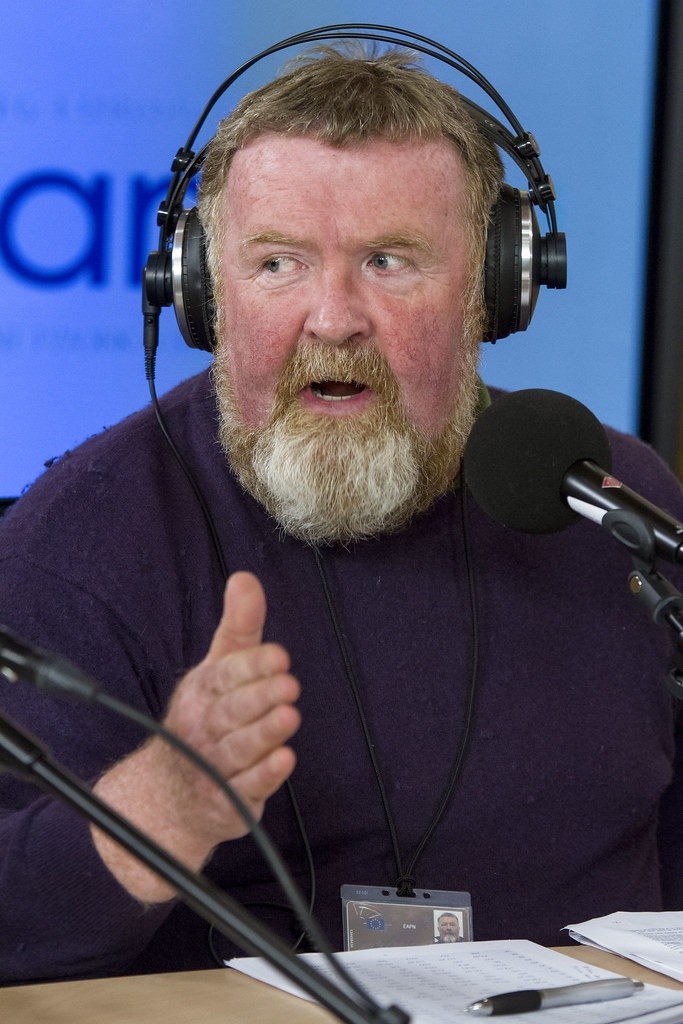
<box><xmin>465</xmin><ymin>388</ymin><xmax>683</xmax><ymax>565</ymax></box>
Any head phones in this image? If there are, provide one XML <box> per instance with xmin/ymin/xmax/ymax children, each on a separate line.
<box><xmin>145</xmin><ymin>22</ymin><xmax>568</xmax><ymax>345</ymax></box>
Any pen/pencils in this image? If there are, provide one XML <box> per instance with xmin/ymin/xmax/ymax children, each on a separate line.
<box><xmin>463</xmin><ymin>977</ymin><xmax>644</xmax><ymax>1016</ymax></box>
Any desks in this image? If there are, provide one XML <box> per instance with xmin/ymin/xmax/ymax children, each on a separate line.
<box><xmin>0</xmin><ymin>945</ymin><xmax>683</xmax><ymax>1024</ymax></box>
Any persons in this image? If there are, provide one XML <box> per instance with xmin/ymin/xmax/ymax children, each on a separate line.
<box><xmin>0</xmin><ymin>38</ymin><xmax>683</xmax><ymax>989</ymax></box>
<box><xmin>434</xmin><ymin>914</ymin><xmax>466</xmax><ymax>942</ymax></box>
